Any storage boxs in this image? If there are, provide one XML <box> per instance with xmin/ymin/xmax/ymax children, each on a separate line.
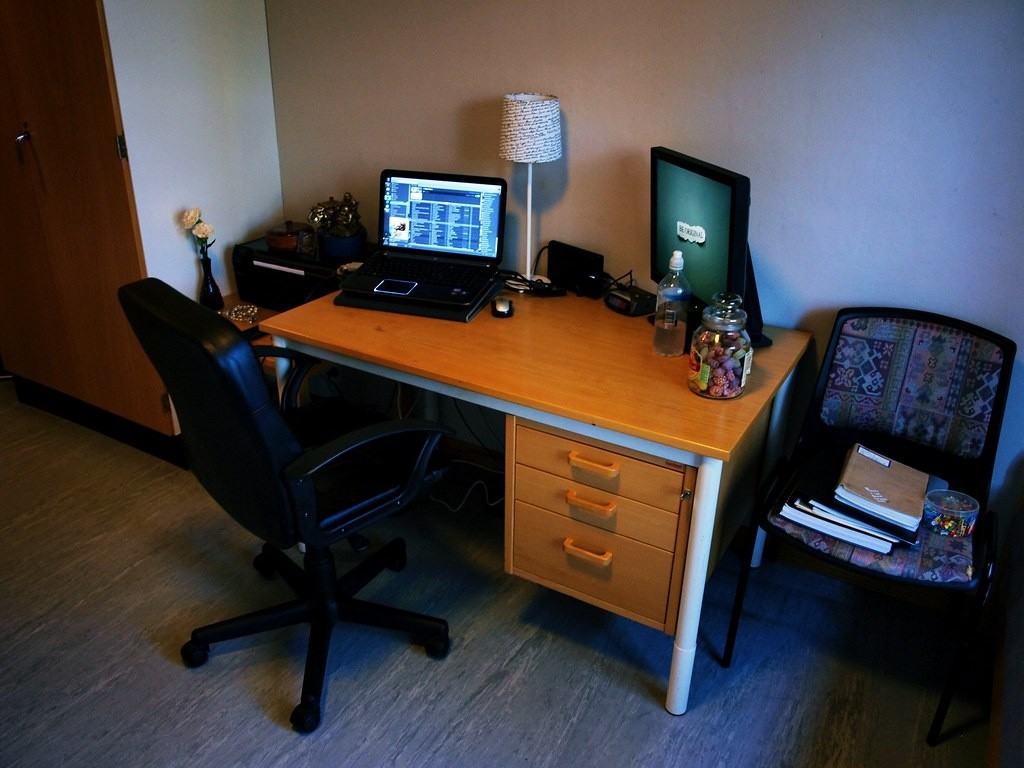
<box><xmin>923</xmin><ymin>489</ymin><xmax>979</xmax><ymax>538</ymax></box>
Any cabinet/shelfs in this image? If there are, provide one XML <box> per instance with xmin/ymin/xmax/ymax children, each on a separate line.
<box><xmin>0</xmin><ymin>0</ymin><xmax>285</xmax><ymax>436</ymax></box>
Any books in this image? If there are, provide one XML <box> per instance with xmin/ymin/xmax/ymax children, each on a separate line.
<box><xmin>777</xmin><ymin>449</ymin><xmax>920</xmax><ymax>556</ymax></box>
<box><xmin>835</xmin><ymin>443</ymin><xmax>929</xmax><ymax>528</ymax></box>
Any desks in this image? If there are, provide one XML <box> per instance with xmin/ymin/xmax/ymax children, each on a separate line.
<box><xmin>258</xmin><ymin>288</ymin><xmax>812</xmax><ymax>716</ymax></box>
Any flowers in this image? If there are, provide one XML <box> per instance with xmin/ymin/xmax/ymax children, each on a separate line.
<box><xmin>182</xmin><ymin>208</ymin><xmax>215</xmax><ymax>258</ymax></box>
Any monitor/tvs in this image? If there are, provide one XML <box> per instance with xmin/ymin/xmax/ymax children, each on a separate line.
<box><xmin>650</xmin><ymin>145</ymin><xmax>773</xmax><ymax>349</ymax></box>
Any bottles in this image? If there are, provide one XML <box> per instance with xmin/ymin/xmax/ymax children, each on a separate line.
<box><xmin>655</xmin><ymin>250</ymin><xmax>688</xmax><ymax>358</ymax></box>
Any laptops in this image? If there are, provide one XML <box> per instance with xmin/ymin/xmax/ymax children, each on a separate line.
<box><xmin>339</xmin><ymin>169</ymin><xmax>507</xmax><ymax>305</ymax></box>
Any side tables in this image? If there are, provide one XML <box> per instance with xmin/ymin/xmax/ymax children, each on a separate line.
<box><xmin>217</xmin><ymin>292</ymin><xmax>332</xmax><ymax>409</ymax></box>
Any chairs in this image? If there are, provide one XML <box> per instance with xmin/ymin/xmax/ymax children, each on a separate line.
<box><xmin>722</xmin><ymin>306</ymin><xmax>1019</xmax><ymax>748</ymax></box>
<box><xmin>116</xmin><ymin>278</ymin><xmax>450</xmax><ymax>735</ymax></box>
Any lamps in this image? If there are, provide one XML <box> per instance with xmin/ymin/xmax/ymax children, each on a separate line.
<box><xmin>498</xmin><ymin>91</ymin><xmax>562</xmax><ymax>293</ymax></box>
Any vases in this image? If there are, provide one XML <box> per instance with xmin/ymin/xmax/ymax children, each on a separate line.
<box><xmin>199</xmin><ymin>258</ymin><xmax>224</xmax><ymax>311</ymax></box>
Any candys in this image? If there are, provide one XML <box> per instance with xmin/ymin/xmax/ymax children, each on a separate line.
<box><xmin>695</xmin><ymin>332</ymin><xmax>749</xmax><ymax>396</ymax></box>
<box><xmin>925</xmin><ymin>496</ymin><xmax>976</xmax><ymax>537</ymax></box>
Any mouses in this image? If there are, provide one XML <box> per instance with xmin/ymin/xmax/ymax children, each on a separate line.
<box><xmin>491</xmin><ymin>297</ymin><xmax>513</xmax><ymax>318</ymax></box>
